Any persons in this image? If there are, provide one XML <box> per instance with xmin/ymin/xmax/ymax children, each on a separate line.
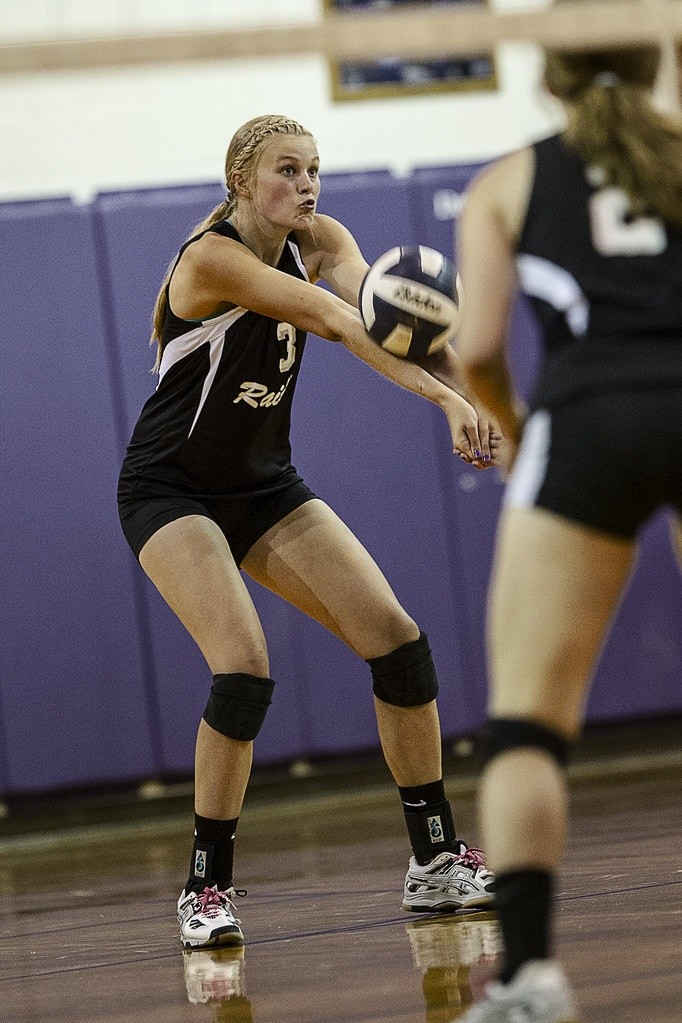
<box><xmin>434</xmin><ymin>0</ymin><xmax>682</xmax><ymax>1020</ymax></box>
<box><xmin>111</xmin><ymin>112</ymin><xmax>522</xmax><ymax>952</ymax></box>
<box><xmin>179</xmin><ymin>917</ymin><xmax>486</xmax><ymax>1023</ymax></box>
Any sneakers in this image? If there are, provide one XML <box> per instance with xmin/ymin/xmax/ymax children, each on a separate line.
<box><xmin>447</xmin><ymin>959</ymin><xmax>581</xmax><ymax>1023</ymax></box>
<box><xmin>176</xmin><ymin>888</ymin><xmax>245</xmax><ymax>950</ymax></box>
<box><xmin>401</xmin><ymin>839</ymin><xmax>496</xmax><ymax>914</ymax></box>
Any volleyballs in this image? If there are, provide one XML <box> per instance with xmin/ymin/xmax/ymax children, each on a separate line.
<box><xmin>359</xmin><ymin>246</ymin><xmax>463</xmax><ymax>358</ymax></box>
